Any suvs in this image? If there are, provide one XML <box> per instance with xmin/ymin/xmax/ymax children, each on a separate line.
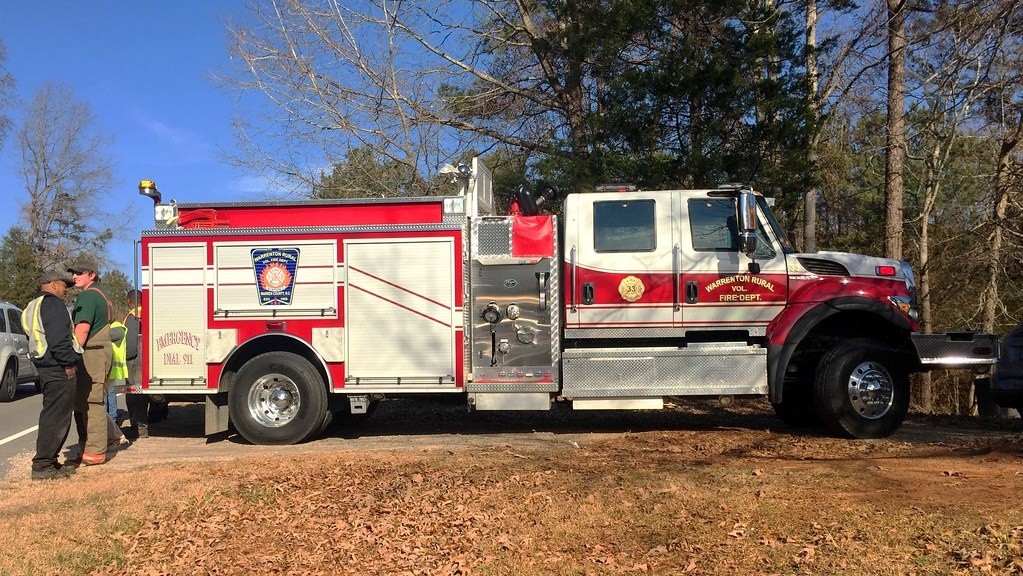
<box><xmin>994</xmin><ymin>320</ymin><xmax>1023</xmax><ymax>417</ymax></box>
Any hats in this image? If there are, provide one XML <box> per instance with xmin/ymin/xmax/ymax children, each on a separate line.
<box><xmin>67</xmin><ymin>259</ymin><xmax>97</xmax><ymax>276</ymax></box>
<box><xmin>42</xmin><ymin>270</ymin><xmax>76</xmax><ymax>288</ymax></box>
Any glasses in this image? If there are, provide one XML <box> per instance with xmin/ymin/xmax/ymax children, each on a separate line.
<box><xmin>72</xmin><ymin>270</ymin><xmax>88</xmax><ymax>276</ymax></box>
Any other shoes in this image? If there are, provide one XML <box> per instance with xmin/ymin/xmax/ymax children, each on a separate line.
<box><xmin>146</xmin><ymin>415</ymin><xmax>160</xmax><ymax>422</ymax></box>
<box><xmin>124</xmin><ymin>429</ymin><xmax>148</xmax><ymax>438</ymax></box>
<box><xmin>107</xmin><ymin>438</ymin><xmax>126</xmax><ymax>451</ymax></box>
<box><xmin>32</xmin><ymin>465</ymin><xmax>70</xmax><ymax>480</ymax></box>
<box><xmin>64</xmin><ymin>456</ymin><xmax>95</xmax><ymax>467</ymax></box>
<box><xmin>54</xmin><ymin>461</ymin><xmax>75</xmax><ymax>474</ymax></box>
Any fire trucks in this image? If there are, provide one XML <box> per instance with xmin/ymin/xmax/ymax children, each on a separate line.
<box><xmin>127</xmin><ymin>156</ymin><xmax>1002</xmax><ymax>442</ymax></box>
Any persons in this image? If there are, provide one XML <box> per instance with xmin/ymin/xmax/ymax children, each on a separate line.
<box><xmin>103</xmin><ymin>321</ymin><xmax>128</xmax><ymax>421</ymax></box>
<box><xmin>122</xmin><ymin>289</ymin><xmax>149</xmax><ymax>438</ymax></box>
<box><xmin>65</xmin><ymin>260</ymin><xmax>131</xmax><ymax>467</ymax></box>
<box><xmin>19</xmin><ymin>269</ymin><xmax>85</xmax><ymax>480</ymax></box>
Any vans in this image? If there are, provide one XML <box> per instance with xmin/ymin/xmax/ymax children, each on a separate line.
<box><xmin>0</xmin><ymin>301</ymin><xmax>46</xmax><ymax>402</ymax></box>
